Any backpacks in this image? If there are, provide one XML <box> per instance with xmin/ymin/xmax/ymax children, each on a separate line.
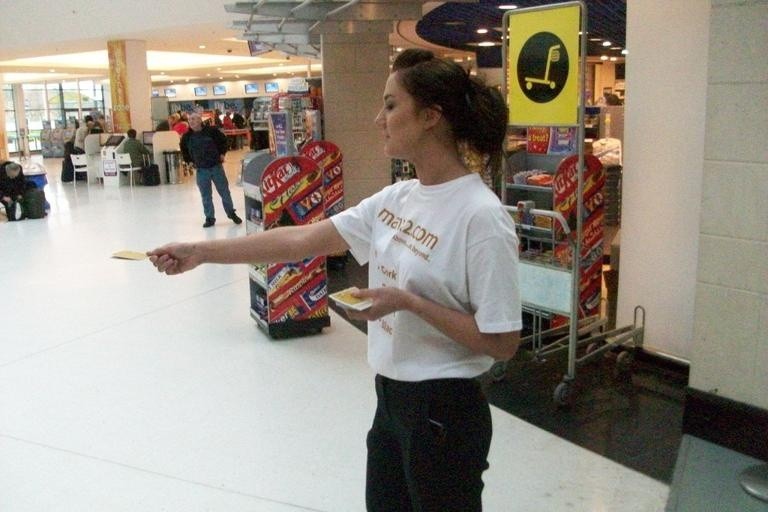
<box><xmin>23</xmin><ymin>189</ymin><xmax>45</xmax><ymax>218</ymax></box>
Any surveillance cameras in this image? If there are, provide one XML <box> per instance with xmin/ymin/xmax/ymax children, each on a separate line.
<box><xmin>227</xmin><ymin>49</ymin><xmax>233</xmax><ymax>53</ymax></box>
<box><xmin>286</xmin><ymin>56</ymin><xmax>290</xmax><ymax>59</ymax></box>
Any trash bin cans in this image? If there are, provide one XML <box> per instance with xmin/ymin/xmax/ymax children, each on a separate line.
<box><xmin>163</xmin><ymin>150</ymin><xmax>183</xmax><ymax>184</ymax></box>
<box><xmin>22</xmin><ymin>159</ymin><xmax>50</xmax><ymax>209</ymax></box>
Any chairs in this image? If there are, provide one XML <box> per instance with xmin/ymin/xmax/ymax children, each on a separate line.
<box><xmin>116</xmin><ymin>153</ymin><xmax>141</xmax><ymax>190</ymax></box>
<box><xmin>70</xmin><ymin>153</ymin><xmax>87</xmax><ymax>187</ymax></box>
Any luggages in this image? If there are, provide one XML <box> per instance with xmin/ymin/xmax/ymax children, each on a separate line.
<box><xmin>142</xmin><ymin>153</ymin><xmax>160</xmax><ymax>185</ymax></box>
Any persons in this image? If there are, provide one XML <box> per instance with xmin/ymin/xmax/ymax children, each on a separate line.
<box><xmin>74</xmin><ymin>116</ymin><xmax>108</xmax><ymax>156</ymax></box>
<box><xmin>123</xmin><ymin>130</ymin><xmax>150</xmax><ymax>184</ymax></box>
<box><xmin>146</xmin><ymin>49</ymin><xmax>522</xmax><ymax>512</ymax></box>
<box><xmin>157</xmin><ymin>109</ymin><xmax>245</xmax><ymax>151</ymax></box>
<box><xmin>181</xmin><ymin>113</ymin><xmax>244</xmax><ymax>227</ymax></box>
<box><xmin>0</xmin><ymin>160</ymin><xmax>37</xmax><ymax>221</ymax></box>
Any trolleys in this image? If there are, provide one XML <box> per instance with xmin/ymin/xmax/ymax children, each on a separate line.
<box><xmin>490</xmin><ymin>0</ymin><xmax>651</xmax><ymax>415</ymax></box>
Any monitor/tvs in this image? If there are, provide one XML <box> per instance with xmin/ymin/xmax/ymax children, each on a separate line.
<box><xmin>245</xmin><ymin>84</ymin><xmax>259</xmax><ymax>94</ymax></box>
<box><xmin>143</xmin><ymin>132</ymin><xmax>156</xmax><ymax>145</ymax></box>
<box><xmin>164</xmin><ymin>88</ymin><xmax>176</xmax><ymax>98</ymax></box>
<box><xmin>152</xmin><ymin>89</ymin><xmax>159</xmax><ymax>96</ymax></box>
<box><xmin>195</xmin><ymin>87</ymin><xmax>207</xmax><ymax>96</ymax></box>
<box><xmin>213</xmin><ymin>85</ymin><xmax>226</xmax><ymax>95</ymax></box>
<box><xmin>265</xmin><ymin>82</ymin><xmax>279</xmax><ymax>92</ymax></box>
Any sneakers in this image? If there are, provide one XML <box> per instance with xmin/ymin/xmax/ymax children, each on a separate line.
<box><xmin>203</xmin><ymin>218</ymin><xmax>215</xmax><ymax>227</ymax></box>
<box><xmin>228</xmin><ymin>213</ymin><xmax>242</xmax><ymax>224</ymax></box>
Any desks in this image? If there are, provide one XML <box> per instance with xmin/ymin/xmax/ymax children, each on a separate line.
<box><xmin>84</xmin><ymin>130</ymin><xmax>182</xmax><ymax>187</ymax></box>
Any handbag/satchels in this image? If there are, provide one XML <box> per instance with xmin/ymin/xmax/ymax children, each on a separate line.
<box><xmin>7</xmin><ymin>199</ymin><xmax>23</xmax><ymax>221</ymax></box>
